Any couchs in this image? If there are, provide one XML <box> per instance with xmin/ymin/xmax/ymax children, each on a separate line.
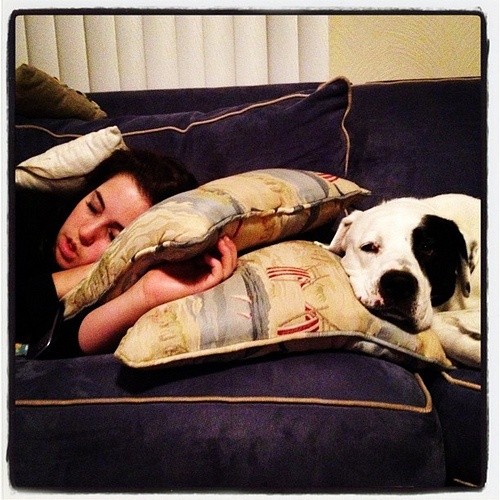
<box><xmin>10</xmin><ymin>76</ymin><xmax>485</xmax><ymax>487</ymax></box>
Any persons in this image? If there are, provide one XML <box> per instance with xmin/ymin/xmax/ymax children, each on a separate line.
<box><xmin>8</xmin><ymin>150</ymin><xmax>241</xmax><ymax>360</ymax></box>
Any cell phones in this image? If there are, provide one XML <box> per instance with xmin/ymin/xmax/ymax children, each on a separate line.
<box><xmin>24</xmin><ymin>310</ymin><xmax>58</xmax><ymax>361</ymax></box>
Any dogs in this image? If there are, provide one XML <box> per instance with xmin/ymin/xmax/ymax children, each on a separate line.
<box><xmin>314</xmin><ymin>194</ymin><xmax>482</xmax><ymax>344</ymax></box>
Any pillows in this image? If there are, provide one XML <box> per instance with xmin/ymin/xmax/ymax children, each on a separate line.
<box><xmin>114</xmin><ymin>238</ymin><xmax>454</xmax><ymax>374</ymax></box>
<box><xmin>60</xmin><ymin>164</ymin><xmax>372</xmax><ymax>321</ymax></box>
<box><xmin>14</xmin><ymin>74</ymin><xmax>354</xmax><ymax>183</ymax></box>
